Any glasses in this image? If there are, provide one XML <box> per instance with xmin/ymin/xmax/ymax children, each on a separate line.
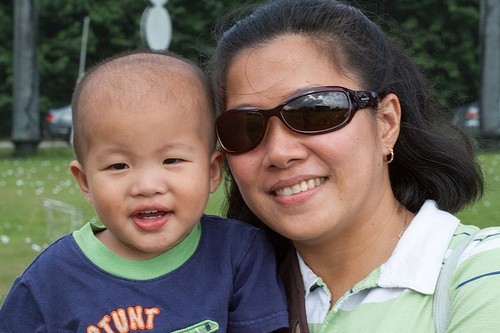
<box><xmin>215</xmin><ymin>86</ymin><xmax>380</xmax><ymax>156</ymax></box>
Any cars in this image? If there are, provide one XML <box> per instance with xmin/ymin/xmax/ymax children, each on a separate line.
<box><xmin>48</xmin><ymin>105</ymin><xmax>76</xmax><ymax>144</ymax></box>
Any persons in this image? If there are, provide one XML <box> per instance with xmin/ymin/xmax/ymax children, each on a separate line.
<box><xmin>0</xmin><ymin>49</ymin><xmax>289</xmax><ymax>333</ymax></box>
<box><xmin>208</xmin><ymin>0</ymin><xmax>500</xmax><ymax>333</ymax></box>
